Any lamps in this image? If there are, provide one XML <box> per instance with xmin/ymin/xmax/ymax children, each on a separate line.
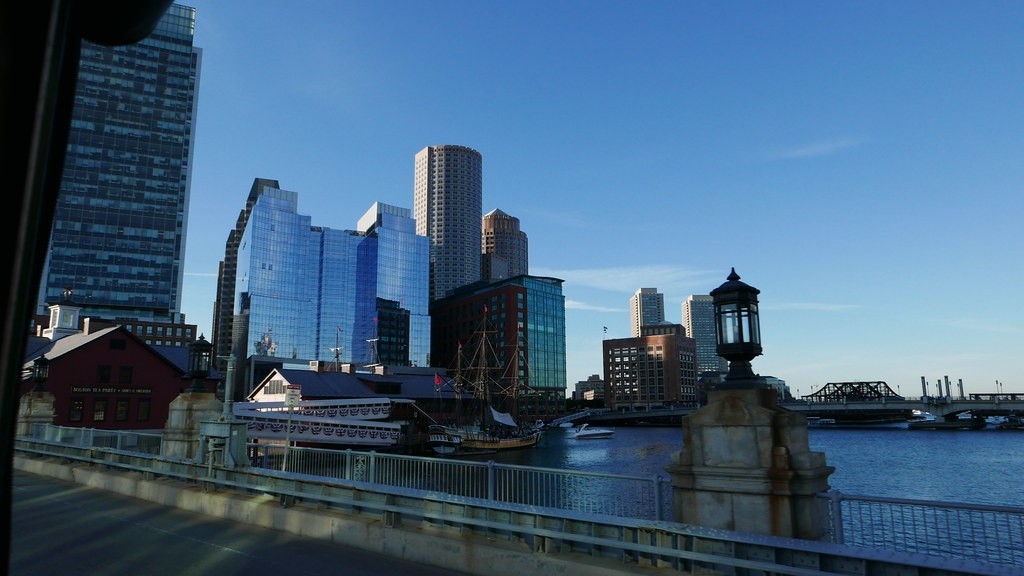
<box><xmin>31</xmin><ymin>351</ymin><xmax>51</xmax><ymax>392</ymax></box>
<box><xmin>709</xmin><ymin>267</ymin><xmax>772</xmax><ymax>389</ymax></box>
<box><xmin>183</xmin><ymin>332</ymin><xmax>215</xmax><ymax>392</ymax></box>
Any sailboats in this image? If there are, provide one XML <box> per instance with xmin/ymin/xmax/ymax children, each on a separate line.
<box><xmin>426</xmin><ymin>303</ymin><xmax>547</xmax><ymax>459</ymax></box>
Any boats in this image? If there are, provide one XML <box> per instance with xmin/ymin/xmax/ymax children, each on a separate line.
<box><xmin>534</xmin><ymin>420</ymin><xmax>550</xmax><ymax>430</ymax></box>
<box><xmin>572</xmin><ymin>423</ymin><xmax>615</xmax><ymax>439</ymax></box>
<box><xmin>807</xmin><ymin>417</ymin><xmax>840</xmax><ymax>428</ymax></box>
